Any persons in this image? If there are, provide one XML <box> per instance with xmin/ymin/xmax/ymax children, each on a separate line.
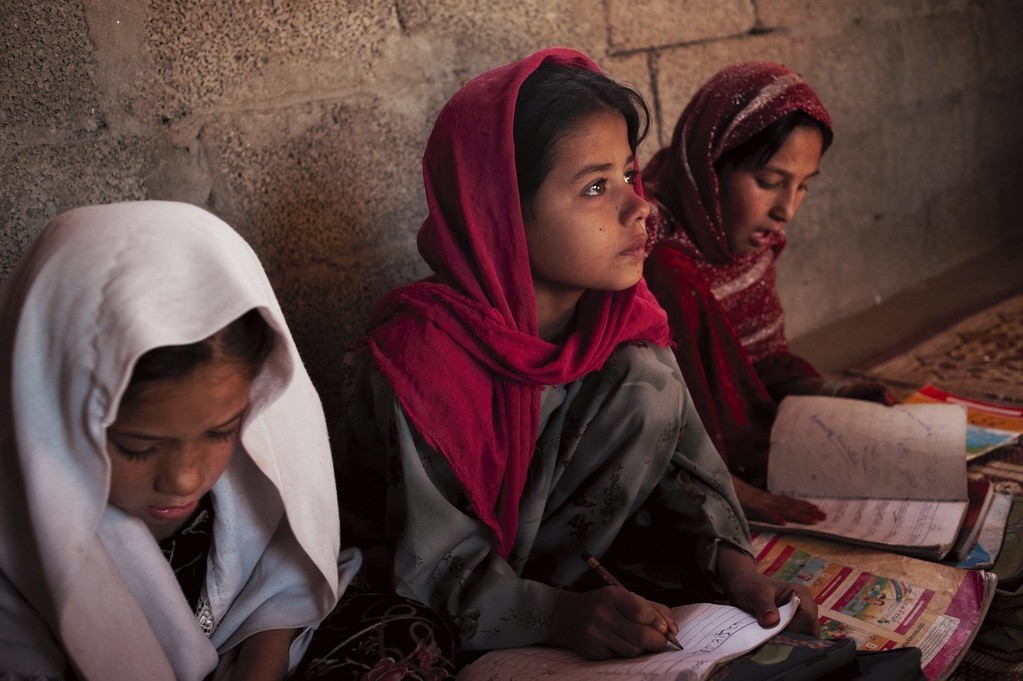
<box><xmin>0</xmin><ymin>201</ymin><xmax>367</xmax><ymax>681</ymax></box>
<box><xmin>322</xmin><ymin>46</ymin><xmax>820</xmax><ymax>681</ymax></box>
<box><xmin>639</xmin><ymin>62</ymin><xmax>901</xmax><ymax>525</ymax></box>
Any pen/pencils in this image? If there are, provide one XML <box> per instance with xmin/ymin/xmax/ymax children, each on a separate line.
<box><xmin>579</xmin><ymin>550</ymin><xmax>684</xmax><ymax>650</ymax></box>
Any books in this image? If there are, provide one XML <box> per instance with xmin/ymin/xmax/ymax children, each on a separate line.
<box><xmin>457</xmin><ymin>384</ymin><xmax>1023</xmax><ymax>681</ymax></box>
